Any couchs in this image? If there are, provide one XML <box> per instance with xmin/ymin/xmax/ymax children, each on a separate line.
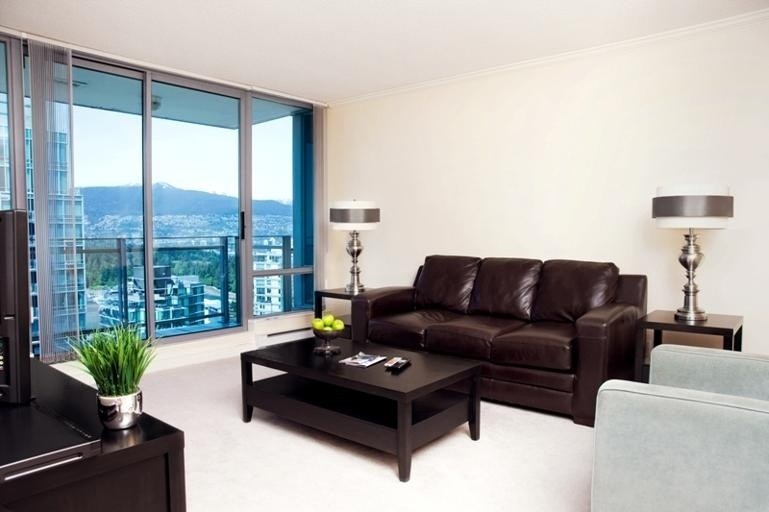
<box><xmin>350</xmin><ymin>254</ymin><xmax>650</xmax><ymax>430</ymax></box>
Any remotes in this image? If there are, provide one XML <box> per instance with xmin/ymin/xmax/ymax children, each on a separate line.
<box><xmin>393</xmin><ymin>359</ymin><xmax>410</xmax><ymax>369</ymax></box>
<box><xmin>384</xmin><ymin>356</ymin><xmax>402</xmax><ymax>368</ymax></box>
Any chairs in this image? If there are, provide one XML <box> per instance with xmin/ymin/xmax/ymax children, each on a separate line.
<box><xmin>589</xmin><ymin>343</ymin><xmax>769</xmax><ymax>512</ymax></box>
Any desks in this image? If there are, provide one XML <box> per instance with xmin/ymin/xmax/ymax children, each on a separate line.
<box><xmin>314</xmin><ymin>287</ymin><xmax>371</xmax><ymax>325</ymax></box>
<box><xmin>635</xmin><ymin>310</ymin><xmax>745</xmax><ymax>389</ymax></box>
<box><xmin>0</xmin><ymin>356</ymin><xmax>187</xmax><ymax>512</ymax></box>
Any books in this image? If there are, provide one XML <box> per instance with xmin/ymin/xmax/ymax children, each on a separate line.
<box><xmin>338</xmin><ymin>351</ymin><xmax>387</xmax><ymax>368</ymax></box>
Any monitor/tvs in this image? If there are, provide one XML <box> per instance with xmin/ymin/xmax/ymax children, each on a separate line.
<box><xmin>0</xmin><ymin>209</ymin><xmax>32</xmax><ymax>407</ymax></box>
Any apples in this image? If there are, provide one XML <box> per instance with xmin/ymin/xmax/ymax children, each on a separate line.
<box><xmin>322</xmin><ymin>327</ymin><xmax>333</xmax><ymax>330</ymax></box>
<box><xmin>322</xmin><ymin>314</ymin><xmax>334</xmax><ymax>326</ymax></box>
<box><xmin>311</xmin><ymin>318</ymin><xmax>324</xmax><ymax>329</ymax></box>
<box><xmin>332</xmin><ymin>320</ymin><xmax>345</xmax><ymax>330</ymax></box>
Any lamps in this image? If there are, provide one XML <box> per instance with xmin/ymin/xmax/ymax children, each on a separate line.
<box><xmin>329</xmin><ymin>204</ymin><xmax>380</xmax><ymax>296</ymax></box>
<box><xmin>653</xmin><ymin>194</ymin><xmax>734</xmax><ymax>320</ymax></box>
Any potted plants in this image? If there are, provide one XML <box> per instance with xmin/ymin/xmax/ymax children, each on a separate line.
<box><xmin>63</xmin><ymin>317</ymin><xmax>155</xmax><ymax>433</ymax></box>
